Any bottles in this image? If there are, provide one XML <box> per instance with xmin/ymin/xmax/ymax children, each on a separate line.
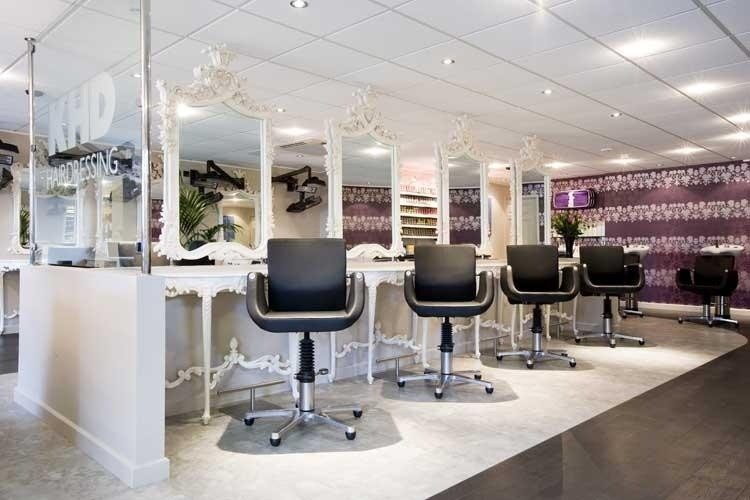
<box><xmin>405</xmin><ymin>242</ymin><xmax>414</xmax><ymax>256</ymax></box>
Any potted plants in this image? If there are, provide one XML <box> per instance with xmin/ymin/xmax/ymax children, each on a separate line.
<box><xmin>549</xmin><ymin>208</ymin><xmax>585</xmax><ymax>258</ymax></box>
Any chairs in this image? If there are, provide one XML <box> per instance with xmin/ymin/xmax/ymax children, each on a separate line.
<box><xmin>675</xmin><ymin>254</ymin><xmax>740</xmax><ymax>329</ymax></box>
<box><xmin>496</xmin><ymin>241</ymin><xmax>580</xmax><ymax>371</ymax></box>
<box><xmin>572</xmin><ymin>243</ymin><xmax>646</xmax><ymax>349</ymax></box>
<box><xmin>617</xmin><ymin>251</ymin><xmax>644</xmax><ymax>319</ymax></box>
<box><xmin>244</xmin><ymin>237</ymin><xmax>365</xmax><ymax>447</ymax></box>
<box><xmin>395</xmin><ymin>243</ymin><xmax>494</xmax><ymax>403</ymax></box>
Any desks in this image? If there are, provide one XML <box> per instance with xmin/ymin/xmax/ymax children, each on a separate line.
<box><xmin>0</xmin><ymin>256</ymin><xmax>19</xmax><ymax>335</ymax></box>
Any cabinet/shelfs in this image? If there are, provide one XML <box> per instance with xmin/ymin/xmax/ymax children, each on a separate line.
<box><xmin>401</xmin><ymin>187</ymin><xmax>439</xmax><ymax>242</ymax></box>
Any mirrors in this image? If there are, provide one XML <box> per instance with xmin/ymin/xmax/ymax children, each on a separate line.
<box><xmin>322</xmin><ymin>84</ymin><xmax>404</xmax><ymax>263</ymax></box>
<box><xmin>436</xmin><ymin>114</ymin><xmax>494</xmax><ymax>258</ymax></box>
<box><xmin>7</xmin><ymin>163</ymin><xmax>29</xmax><ymax>256</ymax></box>
<box><xmin>157</xmin><ymin>41</ymin><xmax>276</xmax><ymax>264</ymax></box>
<box><xmin>35</xmin><ymin>179</ymin><xmax>80</xmax><ymax>248</ymax></box>
<box><xmin>213</xmin><ymin>168</ymin><xmax>260</xmax><ymax>248</ymax></box>
<box><xmin>24</xmin><ymin>0</ymin><xmax>156</xmax><ymax>273</ymax></box>
<box><xmin>92</xmin><ymin>163</ymin><xmax>144</xmax><ymax>255</ymax></box>
<box><xmin>513</xmin><ymin>134</ymin><xmax>554</xmax><ymax>245</ymax></box>
